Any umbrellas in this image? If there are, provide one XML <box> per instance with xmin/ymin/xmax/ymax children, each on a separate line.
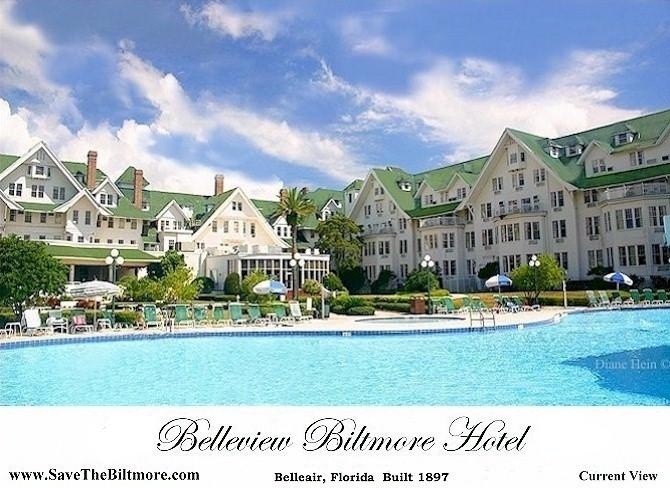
<box><xmin>603</xmin><ymin>270</ymin><xmax>633</xmax><ymax>292</ymax></box>
<box><xmin>485</xmin><ymin>274</ymin><xmax>513</xmax><ymax>295</ymax></box>
<box><xmin>70</xmin><ymin>278</ymin><xmax>122</xmax><ymax>325</ymax></box>
<box><xmin>252</xmin><ymin>279</ymin><xmax>288</xmax><ymax>304</ymax></box>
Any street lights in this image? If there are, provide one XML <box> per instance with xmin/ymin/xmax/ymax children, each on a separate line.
<box><xmin>528</xmin><ymin>253</ymin><xmax>543</xmax><ymax>304</ymax></box>
<box><xmin>288</xmin><ymin>252</ymin><xmax>305</xmax><ymax>301</ymax></box>
<box><xmin>106</xmin><ymin>248</ymin><xmax>124</xmax><ymax>328</ymax></box>
<box><xmin>421</xmin><ymin>254</ymin><xmax>436</xmax><ymax>315</ymax></box>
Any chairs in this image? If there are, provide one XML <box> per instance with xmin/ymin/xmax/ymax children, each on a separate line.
<box><xmin>1</xmin><ymin>297</ymin><xmax>315</xmax><ymax>338</ymax></box>
<box><xmin>585</xmin><ymin>286</ymin><xmax>670</xmax><ymax>306</ymax></box>
<box><xmin>428</xmin><ymin>293</ymin><xmax>542</xmax><ymax>316</ymax></box>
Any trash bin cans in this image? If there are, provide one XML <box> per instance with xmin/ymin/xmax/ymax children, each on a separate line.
<box><xmin>408</xmin><ymin>296</ymin><xmax>426</xmax><ymax>314</ymax></box>
